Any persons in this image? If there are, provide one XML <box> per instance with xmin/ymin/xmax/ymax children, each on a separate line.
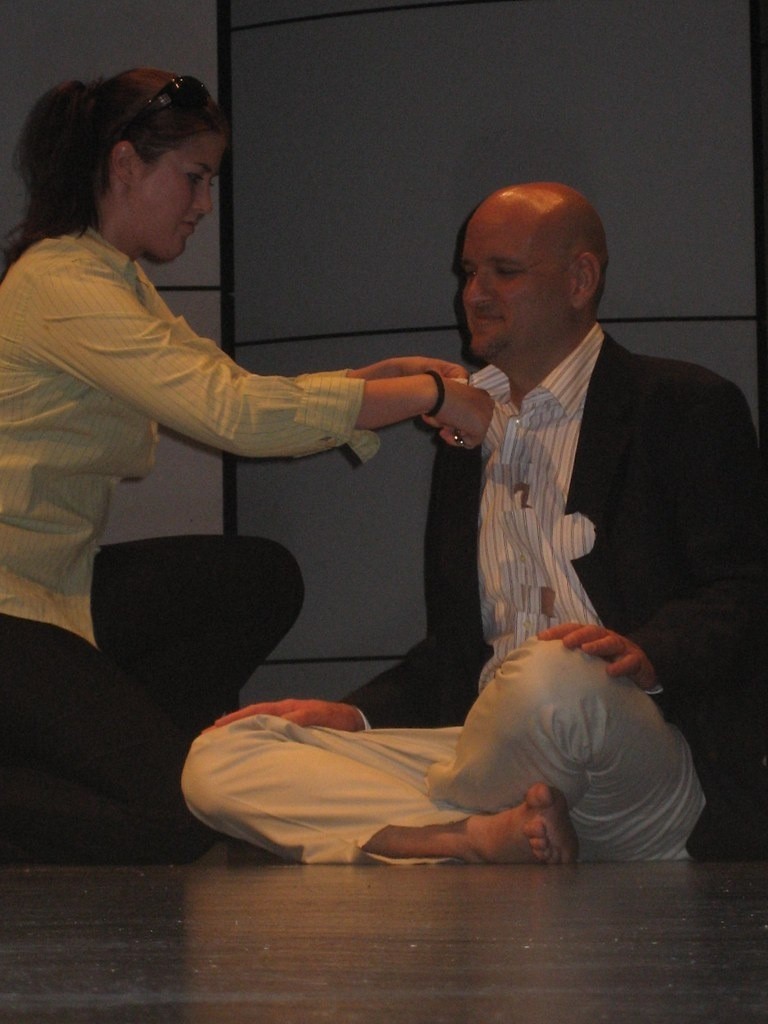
<box><xmin>180</xmin><ymin>181</ymin><xmax>768</xmax><ymax>867</ymax></box>
<box><xmin>0</xmin><ymin>67</ymin><xmax>497</xmax><ymax>862</ymax></box>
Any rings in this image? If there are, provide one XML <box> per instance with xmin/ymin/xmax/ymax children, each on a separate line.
<box><xmin>451</xmin><ymin>428</ymin><xmax>467</xmax><ymax>447</ymax></box>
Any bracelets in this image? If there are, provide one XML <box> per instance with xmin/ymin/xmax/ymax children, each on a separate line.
<box><xmin>425</xmin><ymin>369</ymin><xmax>445</xmax><ymax>417</ymax></box>
